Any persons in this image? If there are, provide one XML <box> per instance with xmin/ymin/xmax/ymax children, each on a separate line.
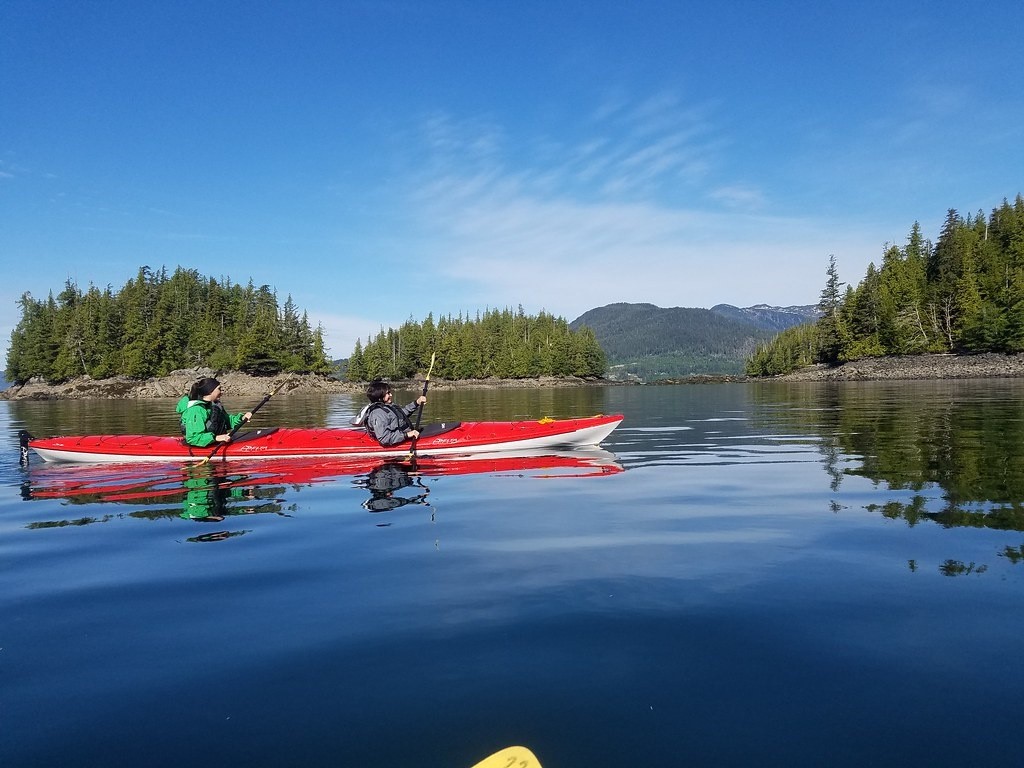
<box><xmin>175</xmin><ymin>377</ymin><xmax>253</xmax><ymax>447</ymax></box>
<box><xmin>351</xmin><ymin>380</ymin><xmax>427</xmax><ymax>446</ymax></box>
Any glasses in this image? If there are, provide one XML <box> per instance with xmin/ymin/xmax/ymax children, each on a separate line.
<box><xmin>385</xmin><ymin>390</ymin><xmax>391</xmax><ymax>395</ymax></box>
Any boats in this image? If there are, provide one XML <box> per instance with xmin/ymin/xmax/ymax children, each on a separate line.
<box><xmin>18</xmin><ymin>413</ymin><xmax>625</xmax><ymax>469</ymax></box>
<box><xmin>21</xmin><ymin>446</ymin><xmax>625</xmax><ymax>503</ymax></box>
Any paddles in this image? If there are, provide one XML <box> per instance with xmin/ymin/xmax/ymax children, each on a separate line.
<box><xmin>173</xmin><ymin>370</ymin><xmax>295</xmax><ymax>470</ymax></box>
<box><xmin>393</xmin><ymin>351</ymin><xmax>439</xmax><ymax>465</ymax></box>
